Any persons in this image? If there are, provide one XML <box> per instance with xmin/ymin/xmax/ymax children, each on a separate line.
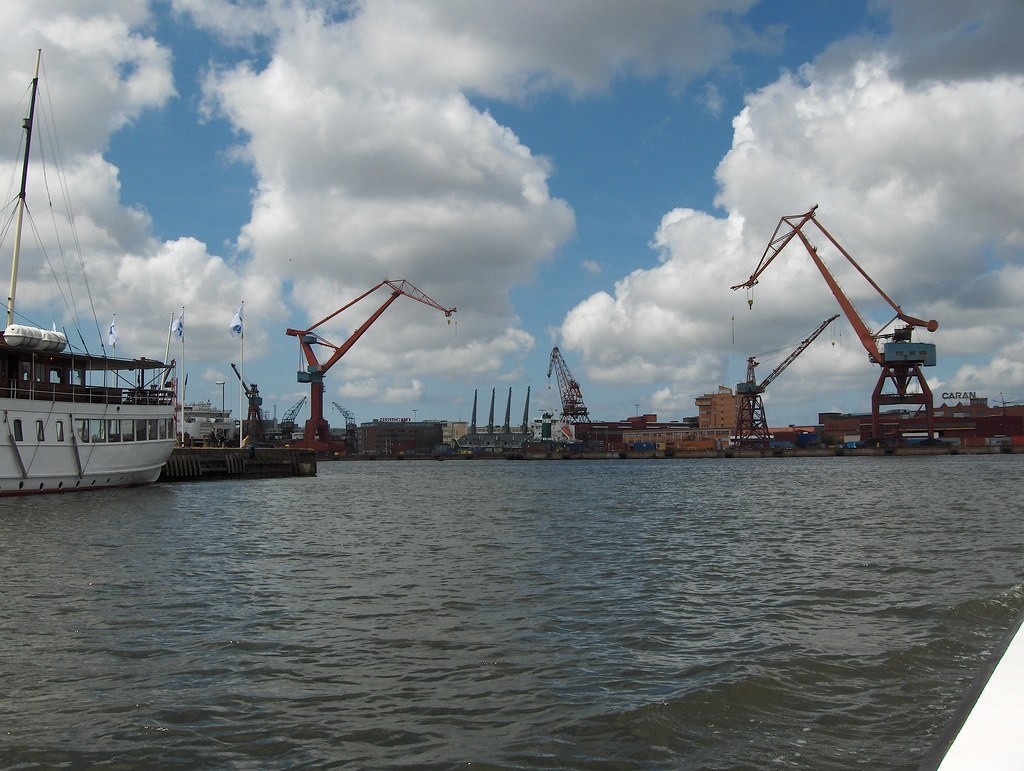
<box><xmin>216</xmin><ymin>428</ymin><xmax>227</xmax><ymax>447</ymax></box>
<box><xmin>243</xmin><ymin>435</ymin><xmax>251</xmax><ymax>448</ymax></box>
<box><xmin>208</xmin><ymin>428</ymin><xmax>221</xmax><ymax>446</ymax></box>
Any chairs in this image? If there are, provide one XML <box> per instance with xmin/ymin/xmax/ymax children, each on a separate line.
<box><xmin>123</xmin><ymin>389</ymin><xmax>175</xmax><ymax>405</ymax></box>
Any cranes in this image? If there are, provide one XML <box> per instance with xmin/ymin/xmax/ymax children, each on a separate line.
<box><xmin>732</xmin><ymin>314</ymin><xmax>840</xmax><ymax>451</ymax></box>
<box><xmin>546</xmin><ymin>346</ymin><xmax>592</xmax><ymax>426</ymax></box>
<box><xmin>281</xmin><ymin>395</ymin><xmax>307</xmax><ymax>440</ymax></box>
<box><xmin>331</xmin><ymin>401</ymin><xmax>359</xmax><ymax>452</ymax></box>
<box><xmin>231</xmin><ymin>362</ymin><xmax>265</xmax><ymax>444</ymax></box>
<box><xmin>729</xmin><ymin>202</ymin><xmax>939</xmax><ymax>448</ymax></box>
<box><xmin>289</xmin><ymin>279</ymin><xmax>458</xmax><ymax>452</ymax></box>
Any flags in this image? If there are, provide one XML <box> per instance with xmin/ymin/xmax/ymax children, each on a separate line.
<box><xmin>229</xmin><ymin>305</ymin><xmax>244</xmax><ymax>338</ymax></box>
<box><xmin>171</xmin><ymin>312</ymin><xmax>184</xmax><ymax>340</ymax></box>
<box><xmin>109</xmin><ymin>321</ymin><xmax>120</xmax><ymax>345</ymax></box>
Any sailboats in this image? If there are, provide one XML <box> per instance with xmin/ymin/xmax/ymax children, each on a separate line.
<box><xmin>1</xmin><ymin>46</ymin><xmax>179</xmax><ymax>496</ymax></box>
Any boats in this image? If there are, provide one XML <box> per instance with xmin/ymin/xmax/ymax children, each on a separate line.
<box><xmin>174</xmin><ymin>399</ymin><xmax>236</xmax><ymax>443</ymax></box>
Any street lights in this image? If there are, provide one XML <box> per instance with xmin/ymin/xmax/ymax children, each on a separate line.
<box><xmin>215</xmin><ymin>381</ymin><xmax>226</xmax><ymax>437</ymax></box>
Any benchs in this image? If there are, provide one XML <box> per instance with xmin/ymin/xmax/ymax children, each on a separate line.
<box><xmin>203</xmin><ymin>435</ymin><xmax>224</xmax><ymax>447</ymax></box>
